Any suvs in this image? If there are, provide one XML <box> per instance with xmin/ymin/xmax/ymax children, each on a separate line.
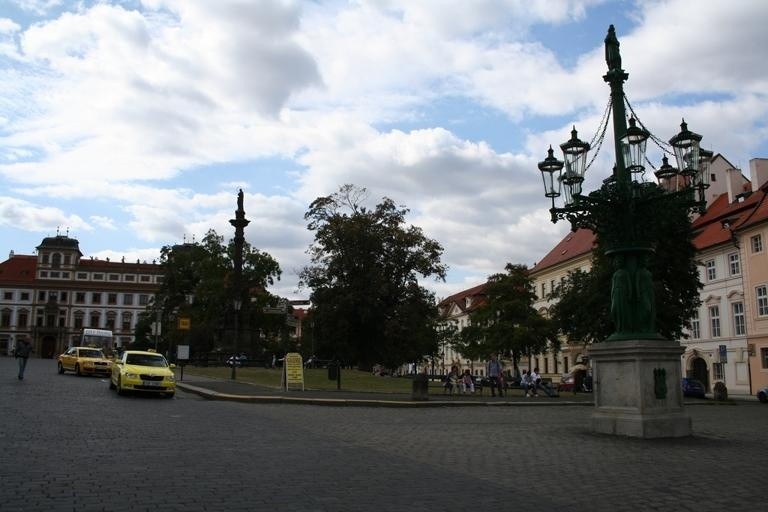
<box><xmin>681</xmin><ymin>377</ymin><xmax>706</xmax><ymax>399</ymax></box>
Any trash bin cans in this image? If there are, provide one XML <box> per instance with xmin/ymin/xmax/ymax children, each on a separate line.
<box><xmin>412</xmin><ymin>376</ymin><xmax>428</xmax><ymax>400</ymax></box>
<box><xmin>328</xmin><ymin>363</ymin><xmax>340</xmax><ymax>389</ymax></box>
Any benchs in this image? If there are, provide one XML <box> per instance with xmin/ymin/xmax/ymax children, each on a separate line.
<box><xmin>445</xmin><ymin>378</ymin><xmax>484</xmax><ymax>396</ymax></box>
<box><xmin>505</xmin><ymin>378</ymin><xmax>549</xmax><ymax>397</ymax></box>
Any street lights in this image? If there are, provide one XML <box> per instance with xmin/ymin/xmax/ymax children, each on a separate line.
<box><xmin>534</xmin><ymin>22</ymin><xmax>713</xmax><ymax>346</ymax></box>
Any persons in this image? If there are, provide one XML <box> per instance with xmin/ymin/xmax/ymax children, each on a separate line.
<box><xmin>15</xmin><ymin>333</ymin><xmax>34</xmax><ymax>379</ymax></box>
<box><xmin>530</xmin><ymin>367</ymin><xmax>555</xmax><ymax>397</ymax></box>
<box><xmin>447</xmin><ymin>366</ymin><xmax>462</xmax><ymax>395</ymax></box>
<box><xmin>460</xmin><ymin>369</ymin><xmax>475</xmax><ymax>393</ymax></box>
<box><xmin>520</xmin><ymin>370</ymin><xmax>539</xmax><ymax>397</ymax></box>
<box><xmin>485</xmin><ymin>352</ymin><xmax>504</xmax><ymax>398</ymax></box>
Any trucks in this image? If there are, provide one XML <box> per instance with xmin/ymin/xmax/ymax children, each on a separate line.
<box><xmin>80</xmin><ymin>326</ymin><xmax>118</xmax><ymax>367</ymax></box>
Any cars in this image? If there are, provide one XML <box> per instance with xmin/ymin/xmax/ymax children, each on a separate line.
<box><xmin>473</xmin><ymin>375</ymin><xmax>520</xmax><ymax>388</ymax></box>
<box><xmin>557</xmin><ymin>374</ymin><xmax>593</xmax><ymax>394</ymax></box>
<box><xmin>108</xmin><ymin>349</ymin><xmax>176</xmax><ymax>399</ymax></box>
<box><xmin>56</xmin><ymin>347</ymin><xmax>115</xmax><ymax>378</ymax></box>
<box><xmin>755</xmin><ymin>386</ymin><xmax>767</xmax><ymax>403</ymax></box>
